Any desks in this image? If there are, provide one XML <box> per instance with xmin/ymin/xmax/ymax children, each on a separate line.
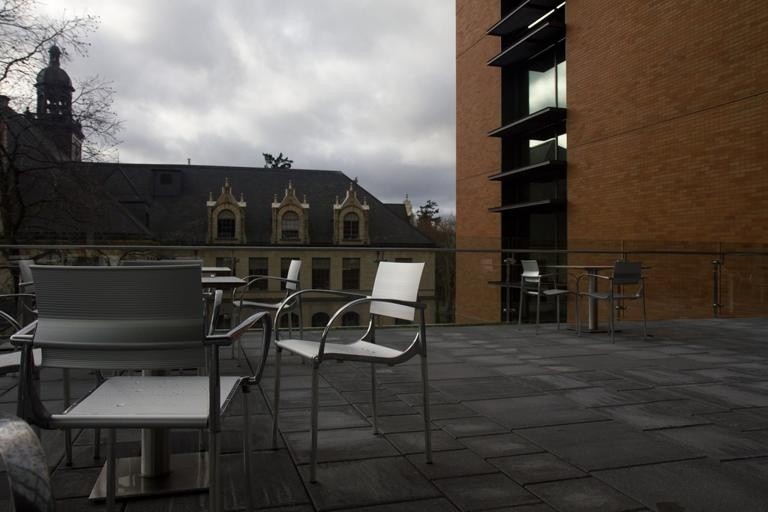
<box><xmin>201</xmin><ymin>266</ymin><xmax>232</xmax><ymax>275</ymax></box>
<box><xmin>89</xmin><ymin>276</ymin><xmax>245</xmax><ymax>498</ymax></box>
<box><xmin>545</xmin><ymin>265</ymin><xmax>651</xmax><ymax>333</ymax></box>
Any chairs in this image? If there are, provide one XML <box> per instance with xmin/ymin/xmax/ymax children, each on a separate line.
<box><xmin>232</xmin><ymin>260</ymin><xmax>305</xmax><ymax>366</ymax></box>
<box><xmin>577</xmin><ymin>261</ymin><xmax>649</xmax><ymax>344</ymax></box>
<box><xmin>272</xmin><ymin>261</ymin><xmax>433</xmax><ymax>482</ymax></box>
<box><xmin>2</xmin><ymin>292</ymin><xmax>75</xmax><ymax>471</ymax></box>
<box><xmin>9</xmin><ymin>262</ymin><xmax>271</xmax><ymax>510</ymax></box>
<box><xmin>18</xmin><ymin>258</ymin><xmax>38</xmax><ymax>316</ymax></box>
<box><xmin>518</xmin><ymin>260</ymin><xmax>578</xmax><ymax>335</ymax></box>
